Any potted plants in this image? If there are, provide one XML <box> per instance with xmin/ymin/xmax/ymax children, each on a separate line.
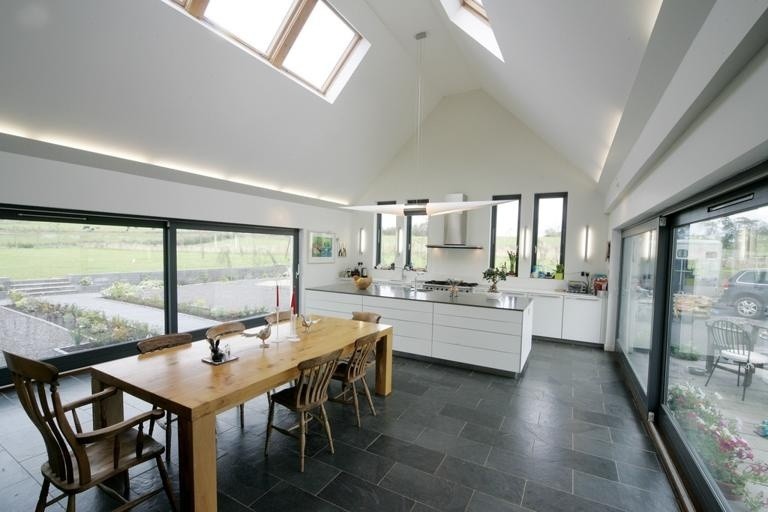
<box><xmin>551</xmin><ymin>263</ymin><xmax>564</xmax><ymax>280</ymax></box>
<box><xmin>506</xmin><ymin>250</ymin><xmax>518</xmax><ymax>277</ymax></box>
<box><xmin>481</xmin><ymin>267</ymin><xmax>506</xmax><ymax>300</ymax></box>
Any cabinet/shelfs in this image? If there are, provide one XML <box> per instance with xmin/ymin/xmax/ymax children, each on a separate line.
<box><xmin>526</xmin><ymin>294</ymin><xmax>602</xmax><ymax>346</ymax></box>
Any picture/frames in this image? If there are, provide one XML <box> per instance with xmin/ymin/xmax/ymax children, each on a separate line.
<box><xmin>307</xmin><ymin>232</ymin><xmax>336</xmax><ymax>264</ymax></box>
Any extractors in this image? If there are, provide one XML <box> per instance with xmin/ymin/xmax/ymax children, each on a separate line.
<box><xmin>425</xmin><ymin>193</ymin><xmax>486</xmax><ymax>249</ymax></box>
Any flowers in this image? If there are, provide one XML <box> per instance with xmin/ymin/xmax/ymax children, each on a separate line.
<box><xmin>665</xmin><ymin>381</ymin><xmax>767</xmax><ymax>512</ymax></box>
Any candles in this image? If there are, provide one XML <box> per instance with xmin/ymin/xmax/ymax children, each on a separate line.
<box><xmin>290</xmin><ymin>285</ymin><xmax>297</xmax><ymax>314</ymax></box>
<box><xmin>276</xmin><ymin>284</ymin><xmax>280</xmax><ymax>306</ymax></box>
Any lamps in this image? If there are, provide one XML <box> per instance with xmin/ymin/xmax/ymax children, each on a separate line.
<box><xmin>337</xmin><ymin>32</ymin><xmax>514</xmax><ymax>218</ymax></box>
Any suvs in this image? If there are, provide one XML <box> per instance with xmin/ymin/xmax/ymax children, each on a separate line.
<box><xmin>718</xmin><ymin>268</ymin><xmax>768</xmax><ymax>319</ymax></box>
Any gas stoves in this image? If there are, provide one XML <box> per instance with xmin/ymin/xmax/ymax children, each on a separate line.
<box><xmin>421</xmin><ymin>280</ymin><xmax>479</xmax><ymax>294</ymax></box>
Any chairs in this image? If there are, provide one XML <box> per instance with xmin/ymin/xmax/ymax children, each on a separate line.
<box><xmin>704</xmin><ymin>319</ymin><xmax>768</xmax><ymax>402</ymax></box>
<box><xmin>2</xmin><ymin>350</ymin><xmax>179</xmax><ymax>512</ymax></box>
<box><xmin>137</xmin><ymin>310</ymin><xmax>380</xmax><ymax>472</ymax></box>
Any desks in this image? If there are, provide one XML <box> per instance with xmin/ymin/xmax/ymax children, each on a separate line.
<box><xmin>89</xmin><ymin>315</ymin><xmax>395</xmax><ymax>511</ymax></box>
<box><xmin>705</xmin><ymin>321</ymin><xmax>745</xmax><ymax>375</ymax></box>
<box><xmin>741</xmin><ymin>322</ymin><xmax>768</xmax><ymax>387</ymax></box>
